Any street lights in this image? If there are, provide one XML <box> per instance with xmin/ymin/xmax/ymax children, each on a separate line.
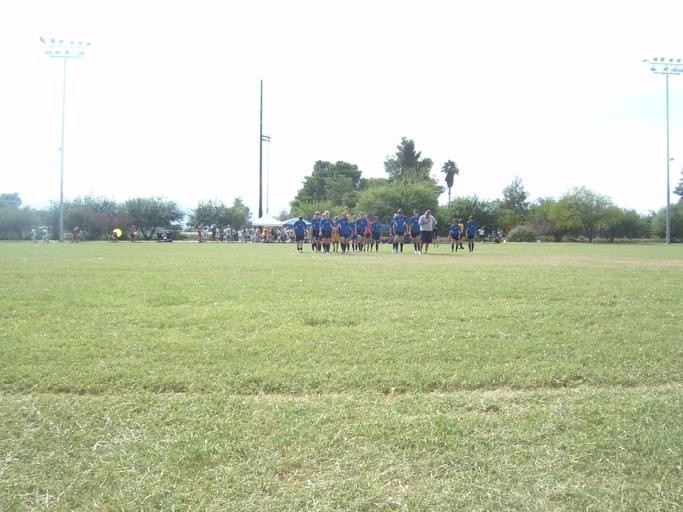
<box><xmin>639</xmin><ymin>56</ymin><xmax>682</xmax><ymax>244</ymax></box>
<box><xmin>37</xmin><ymin>34</ymin><xmax>94</xmax><ymax>240</ymax></box>
<box><xmin>260</xmin><ymin>134</ymin><xmax>272</xmax><ymax>212</ymax></box>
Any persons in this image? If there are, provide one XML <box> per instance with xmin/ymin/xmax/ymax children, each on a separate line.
<box><xmin>130</xmin><ymin>225</ymin><xmax>136</xmax><ymax>242</ymax></box>
<box><xmin>112</xmin><ymin>232</ymin><xmax>118</xmax><ymax>242</ymax></box>
<box><xmin>31</xmin><ymin>226</ymin><xmax>48</xmax><ymax>243</ymax></box>
<box><xmin>71</xmin><ymin>225</ymin><xmax>79</xmax><ymax>244</ymax></box>
<box><xmin>162</xmin><ymin>231</ymin><xmax>167</xmax><ymax>241</ymax></box>
